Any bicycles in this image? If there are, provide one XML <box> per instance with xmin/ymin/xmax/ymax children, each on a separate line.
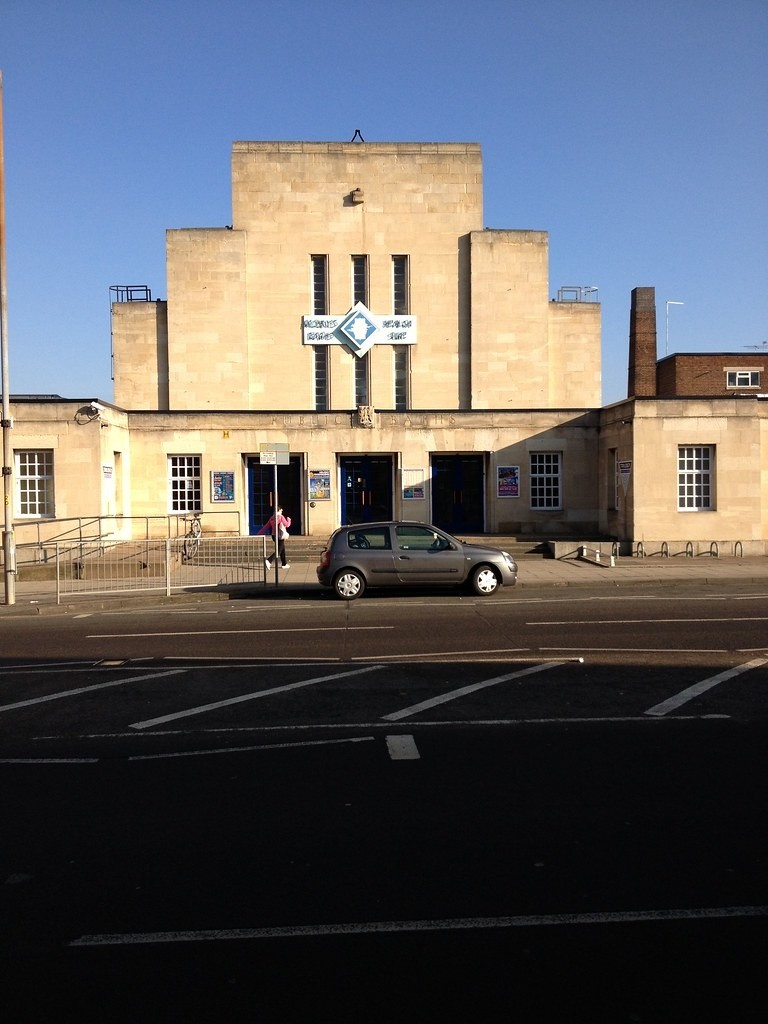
<box><xmin>178</xmin><ymin>511</ymin><xmax>204</xmax><ymax>559</ymax></box>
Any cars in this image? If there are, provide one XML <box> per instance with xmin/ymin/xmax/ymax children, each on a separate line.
<box><xmin>316</xmin><ymin>519</ymin><xmax>518</xmax><ymax>598</ymax></box>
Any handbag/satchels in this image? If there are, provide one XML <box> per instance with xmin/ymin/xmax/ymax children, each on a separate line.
<box><xmin>279</xmin><ymin>521</ymin><xmax>290</xmax><ymax>540</ymax></box>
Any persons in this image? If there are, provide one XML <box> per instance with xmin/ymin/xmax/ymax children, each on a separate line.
<box><xmin>257</xmin><ymin>504</ymin><xmax>291</xmax><ymax>571</ymax></box>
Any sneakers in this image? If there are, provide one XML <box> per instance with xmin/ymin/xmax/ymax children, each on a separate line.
<box><xmin>265</xmin><ymin>560</ymin><xmax>271</xmax><ymax>571</ymax></box>
<box><xmin>281</xmin><ymin>564</ymin><xmax>290</xmax><ymax>569</ymax></box>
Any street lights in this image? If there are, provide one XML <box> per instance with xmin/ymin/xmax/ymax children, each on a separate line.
<box><xmin>666</xmin><ymin>300</ymin><xmax>684</xmax><ymax>356</ymax></box>
